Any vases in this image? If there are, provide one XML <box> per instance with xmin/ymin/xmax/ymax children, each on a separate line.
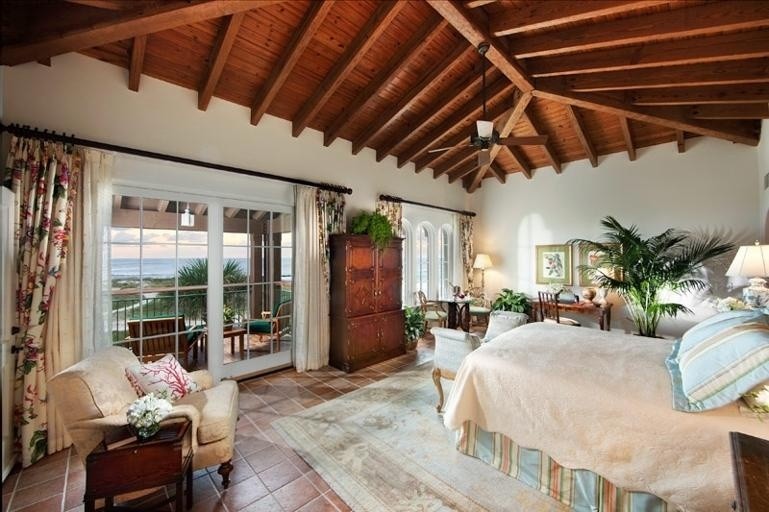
<box><xmin>136</xmin><ymin>422</ymin><xmax>160</xmax><ymax>442</ymax></box>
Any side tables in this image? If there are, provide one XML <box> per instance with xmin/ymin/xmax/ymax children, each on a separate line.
<box><xmin>200</xmin><ymin>326</ymin><xmax>247</xmax><ymax>361</ymax></box>
<box><xmin>442</xmin><ymin>296</ymin><xmax>475</xmax><ymax>333</ymax></box>
<box><xmin>84</xmin><ymin>421</ymin><xmax>194</xmax><ymax>512</ymax></box>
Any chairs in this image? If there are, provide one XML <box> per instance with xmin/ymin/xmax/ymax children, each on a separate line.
<box><xmin>429</xmin><ymin>327</ymin><xmax>480</xmax><ymax>413</ymax></box>
<box><xmin>241</xmin><ymin>299</ymin><xmax>292</xmax><ymax>353</ymax></box>
<box><xmin>125</xmin><ymin>314</ymin><xmax>204</xmax><ymax>371</ymax></box>
<box><xmin>538</xmin><ymin>291</ymin><xmax>581</xmax><ymax>327</ymax></box>
<box><xmin>418</xmin><ymin>290</ymin><xmax>447</xmax><ymax>339</ymax></box>
<box><xmin>465</xmin><ymin>287</ymin><xmax>492</xmax><ymax>333</ymax></box>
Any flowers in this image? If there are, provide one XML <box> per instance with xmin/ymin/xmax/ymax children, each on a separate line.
<box><xmin>127</xmin><ymin>393</ymin><xmax>172</xmax><ymax>428</ymax></box>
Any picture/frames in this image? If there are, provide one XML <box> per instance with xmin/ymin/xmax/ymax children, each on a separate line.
<box><xmin>579</xmin><ymin>244</ymin><xmax>623</xmax><ymax>288</ymax></box>
<box><xmin>535</xmin><ymin>244</ymin><xmax>572</xmax><ymax>286</ymax></box>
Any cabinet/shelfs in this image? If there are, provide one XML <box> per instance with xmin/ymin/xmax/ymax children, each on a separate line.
<box><xmin>329</xmin><ymin>233</ymin><xmax>406</xmax><ymax>374</ymax></box>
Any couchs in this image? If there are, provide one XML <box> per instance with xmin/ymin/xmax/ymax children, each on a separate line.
<box><xmin>47</xmin><ymin>346</ymin><xmax>239</xmax><ymax>488</ymax></box>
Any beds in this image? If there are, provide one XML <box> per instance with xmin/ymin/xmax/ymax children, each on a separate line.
<box><xmin>442</xmin><ymin>321</ymin><xmax>769</xmax><ymax>511</ymax></box>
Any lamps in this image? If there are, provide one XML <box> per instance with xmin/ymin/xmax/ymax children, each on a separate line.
<box><xmin>724</xmin><ymin>242</ymin><xmax>768</xmax><ymax>307</ymax></box>
<box><xmin>467</xmin><ymin>120</ymin><xmax>499</xmax><ymax>144</ymax></box>
<box><xmin>473</xmin><ymin>254</ymin><xmax>492</xmax><ymax>326</ymax></box>
<box><xmin>180</xmin><ymin>202</ymin><xmax>196</xmax><ymax>227</ymax></box>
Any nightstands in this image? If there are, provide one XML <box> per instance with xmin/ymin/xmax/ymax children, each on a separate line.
<box><xmin>728</xmin><ymin>431</ymin><xmax>768</xmax><ymax>512</ymax></box>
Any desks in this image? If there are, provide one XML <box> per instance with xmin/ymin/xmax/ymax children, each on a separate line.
<box><xmin>531</xmin><ymin>299</ymin><xmax>614</xmax><ymax>331</ymax></box>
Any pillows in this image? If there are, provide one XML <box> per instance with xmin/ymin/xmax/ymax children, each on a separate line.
<box><xmin>125</xmin><ymin>354</ymin><xmax>202</xmax><ymax>402</ymax></box>
<box><xmin>665</xmin><ymin>310</ymin><xmax>768</xmax><ymax>412</ymax></box>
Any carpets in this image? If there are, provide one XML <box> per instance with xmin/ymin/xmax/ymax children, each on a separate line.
<box><xmin>269</xmin><ymin>361</ymin><xmax>578</xmax><ymax>511</ymax></box>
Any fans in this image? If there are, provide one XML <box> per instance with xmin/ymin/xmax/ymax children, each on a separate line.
<box><xmin>428</xmin><ymin>42</ymin><xmax>549</xmax><ymax>167</ymax></box>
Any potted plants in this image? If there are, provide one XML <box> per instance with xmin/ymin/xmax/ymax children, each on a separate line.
<box><xmin>405</xmin><ymin>306</ymin><xmax>425</xmax><ymax>351</ymax></box>
<box><xmin>224</xmin><ymin>304</ymin><xmax>235</xmax><ymax>331</ymax></box>
<box><xmin>565</xmin><ymin>215</ymin><xmax>736</xmax><ymax>339</ymax></box>
<box><xmin>350</xmin><ymin>209</ymin><xmax>392</xmax><ymax>249</ymax></box>
